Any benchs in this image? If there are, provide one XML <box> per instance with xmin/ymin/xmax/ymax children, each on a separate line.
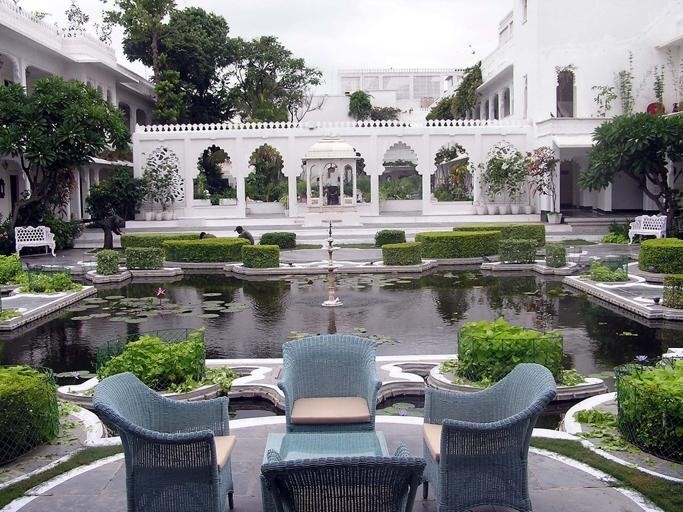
<box><xmin>628</xmin><ymin>215</ymin><xmax>667</xmax><ymax>245</ymax></box>
<box><xmin>15</xmin><ymin>226</ymin><xmax>56</xmax><ymax>257</ymax></box>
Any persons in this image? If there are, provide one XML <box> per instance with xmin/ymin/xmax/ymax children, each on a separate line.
<box><xmin>234</xmin><ymin>226</ymin><xmax>254</xmax><ymax>245</ymax></box>
<box><xmin>200</xmin><ymin>231</ymin><xmax>216</xmax><ymax>239</ymax></box>
<box><xmin>323</xmin><ymin>167</ymin><xmax>340</xmax><ymax>205</ymax></box>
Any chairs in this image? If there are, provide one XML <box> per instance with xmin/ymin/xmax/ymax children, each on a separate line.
<box><xmin>260</xmin><ymin>445</ymin><xmax>428</xmax><ymax>512</ymax></box>
<box><xmin>277</xmin><ymin>335</ymin><xmax>382</xmax><ymax>432</ymax></box>
<box><xmin>93</xmin><ymin>371</ymin><xmax>237</xmax><ymax>512</ymax></box>
<box><xmin>422</xmin><ymin>363</ymin><xmax>557</xmax><ymax>511</ymax></box>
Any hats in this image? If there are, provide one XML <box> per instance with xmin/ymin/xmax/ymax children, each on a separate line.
<box><xmin>234</xmin><ymin>226</ymin><xmax>243</xmax><ymax>232</ymax></box>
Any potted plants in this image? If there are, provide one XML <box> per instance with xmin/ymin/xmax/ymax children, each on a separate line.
<box><xmin>140</xmin><ymin>145</ymin><xmax>184</xmax><ymax>220</ymax></box>
<box><xmin>474</xmin><ymin>138</ymin><xmax>563</xmax><ymax>224</ymax></box>
